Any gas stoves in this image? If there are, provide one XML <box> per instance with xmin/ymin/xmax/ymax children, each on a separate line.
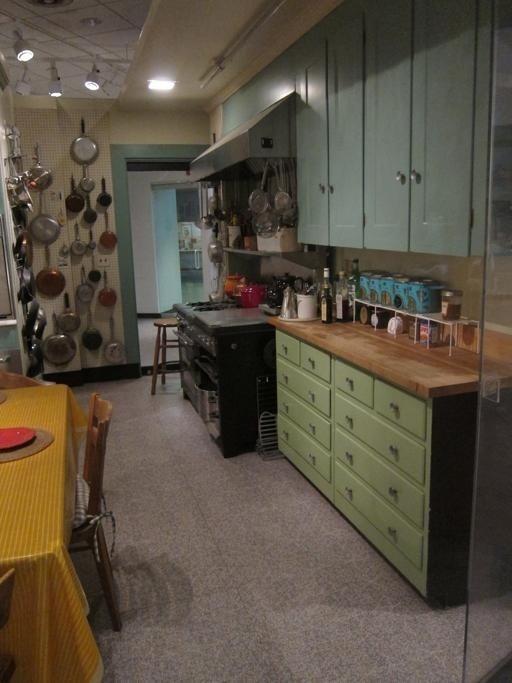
<box><xmin>172</xmin><ymin>300</ymin><xmax>274</xmax><ymax>352</ymax></box>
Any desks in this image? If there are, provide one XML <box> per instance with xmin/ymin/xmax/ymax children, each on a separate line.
<box><xmin>0</xmin><ymin>383</ymin><xmax>105</xmax><ymax>683</ymax></box>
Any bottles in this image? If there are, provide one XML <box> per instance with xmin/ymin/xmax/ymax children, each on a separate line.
<box><xmin>349</xmin><ymin>257</ymin><xmax>362</xmax><ymax>301</ymax></box>
<box><xmin>319</xmin><ymin>267</ymin><xmax>332</xmax><ymax>323</ymax></box>
<box><xmin>335</xmin><ymin>271</ymin><xmax>350</xmax><ymax>321</ymax></box>
<box><xmin>439</xmin><ymin>288</ymin><xmax>463</xmax><ymax>320</ymax></box>
<box><xmin>370</xmin><ymin>310</ymin><xmax>386</xmax><ymax>329</ymax></box>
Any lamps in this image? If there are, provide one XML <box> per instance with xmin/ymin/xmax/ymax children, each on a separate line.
<box><xmin>145</xmin><ymin>62</ymin><xmax>179</xmax><ymax>91</ymax></box>
<box><xmin>8</xmin><ymin>26</ymin><xmax>101</xmax><ymax>98</ymax></box>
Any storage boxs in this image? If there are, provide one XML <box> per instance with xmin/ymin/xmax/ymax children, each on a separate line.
<box><xmin>256</xmin><ymin>225</ymin><xmax>303</xmax><ymax>253</ymax></box>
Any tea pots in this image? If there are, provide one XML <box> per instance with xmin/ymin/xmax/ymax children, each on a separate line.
<box><xmin>267</xmin><ymin>274</ymin><xmax>307</xmax><ymax>308</ymax></box>
<box><xmin>238</xmin><ymin>280</ymin><xmax>266</xmax><ymax>307</ymax></box>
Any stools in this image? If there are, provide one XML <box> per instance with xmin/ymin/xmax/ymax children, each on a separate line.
<box><xmin>150</xmin><ymin>317</ymin><xmax>178</xmax><ymax>395</ymax></box>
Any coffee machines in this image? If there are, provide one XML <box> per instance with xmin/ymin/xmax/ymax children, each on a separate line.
<box><xmin>206</xmin><ymin>264</ymin><xmax>225</xmax><ymax>302</ymax></box>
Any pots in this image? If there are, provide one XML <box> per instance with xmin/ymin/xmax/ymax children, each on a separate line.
<box><xmin>55</xmin><ymin>113</ymin><xmax>127</xmax><ymax>369</ymax></box>
<box><xmin>208</xmin><ymin>222</ymin><xmax>223</xmax><ymax>264</ymax></box>
<box><xmin>2</xmin><ymin>125</ymin><xmax>81</xmax><ymax>368</ymax></box>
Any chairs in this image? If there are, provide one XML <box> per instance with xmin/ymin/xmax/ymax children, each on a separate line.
<box><xmin>0</xmin><ymin>372</ymin><xmax>45</xmax><ymax>389</ymax></box>
<box><xmin>0</xmin><ymin>568</ymin><xmax>17</xmax><ymax>683</ymax></box>
<box><xmin>69</xmin><ymin>392</ymin><xmax>123</xmax><ymax>633</ymax></box>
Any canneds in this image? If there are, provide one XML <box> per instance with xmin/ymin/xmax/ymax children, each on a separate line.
<box><xmin>224</xmin><ymin>276</ymin><xmax>241</xmax><ymax>298</ymax></box>
<box><xmin>359</xmin><ymin>271</ymin><xmax>462</xmax><ymax>321</ymax></box>
<box><xmin>420</xmin><ymin>322</ymin><xmax>437</xmax><ymax>345</ymax></box>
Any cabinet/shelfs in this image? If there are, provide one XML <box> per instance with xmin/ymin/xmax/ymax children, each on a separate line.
<box><xmin>276</xmin><ymin>327</ymin><xmax>478</xmax><ymax>599</ymax></box>
<box><xmin>295</xmin><ymin>0</ymin><xmax>512</xmax><ymax>260</ymax></box>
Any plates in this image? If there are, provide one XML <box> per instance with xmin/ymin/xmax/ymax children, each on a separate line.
<box><xmin>0</xmin><ymin>425</ymin><xmax>36</xmax><ymax>453</ymax></box>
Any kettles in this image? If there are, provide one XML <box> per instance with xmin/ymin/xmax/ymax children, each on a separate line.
<box><xmin>279</xmin><ymin>286</ymin><xmax>298</xmax><ymax>319</ymax></box>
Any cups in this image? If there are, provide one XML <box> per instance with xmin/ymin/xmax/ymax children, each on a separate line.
<box><xmin>295</xmin><ymin>291</ymin><xmax>318</xmax><ymax>319</ymax></box>
